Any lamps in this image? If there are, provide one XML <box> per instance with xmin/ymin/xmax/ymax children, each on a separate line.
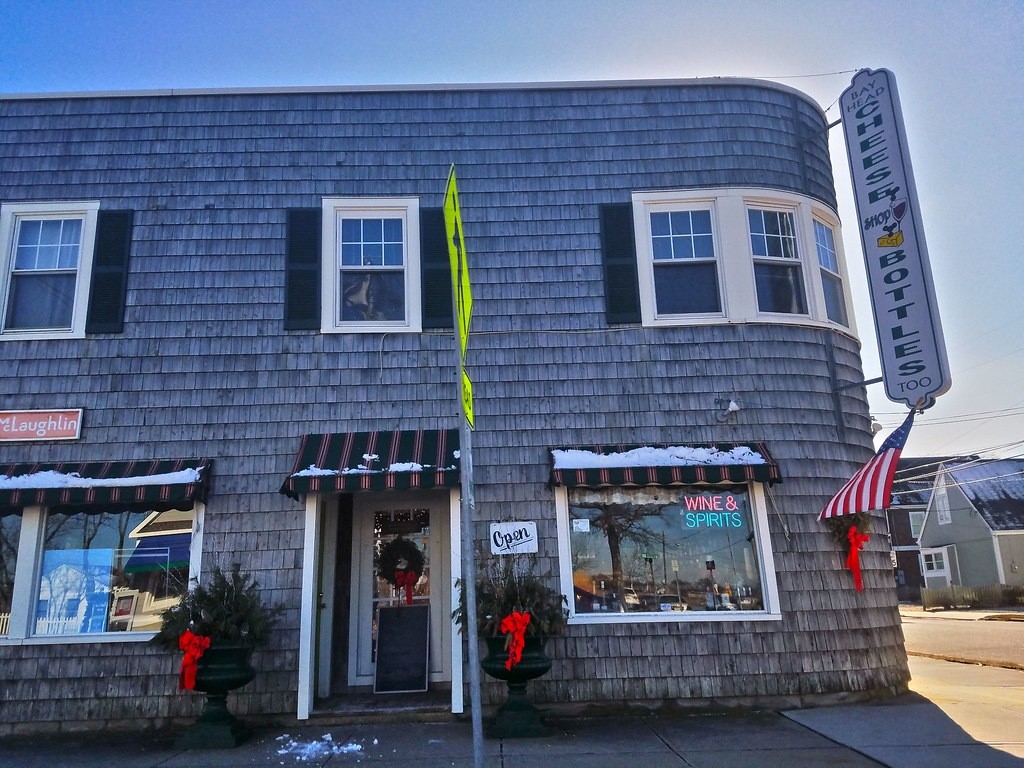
<box><xmin>872</xmin><ymin>423</ymin><xmax>883</xmax><ymax>440</ymax></box>
<box><xmin>715</xmin><ymin>397</ymin><xmax>740</xmax><ymax>423</ymax></box>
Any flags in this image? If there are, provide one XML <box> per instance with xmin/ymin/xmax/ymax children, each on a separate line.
<box><xmin>817</xmin><ymin>408</ymin><xmax>916</xmax><ymax>522</ymax></box>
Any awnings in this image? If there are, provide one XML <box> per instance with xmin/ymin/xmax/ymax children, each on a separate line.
<box><xmin>279</xmin><ymin>429</ymin><xmax>462</xmax><ymax>501</ymax></box>
<box><xmin>0</xmin><ymin>459</ymin><xmax>215</xmax><ymax>512</ymax></box>
<box><xmin>546</xmin><ymin>443</ymin><xmax>783</xmax><ymax>493</ymax></box>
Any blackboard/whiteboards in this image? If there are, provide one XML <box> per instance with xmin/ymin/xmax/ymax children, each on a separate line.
<box><xmin>374</xmin><ymin>604</ymin><xmax>429</xmax><ymax>693</ymax></box>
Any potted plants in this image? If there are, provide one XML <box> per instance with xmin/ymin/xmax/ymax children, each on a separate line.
<box><xmin>450</xmin><ymin>539</ymin><xmax>571</xmax><ymax>739</ymax></box>
<box><xmin>821</xmin><ymin>510</ymin><xmax>871</xmax><ymax>559</ymax></box>
<box><xmin>149</xmin><ymin>558</ymin><xmax>287</xmax><ymax>747</ymax></box>
